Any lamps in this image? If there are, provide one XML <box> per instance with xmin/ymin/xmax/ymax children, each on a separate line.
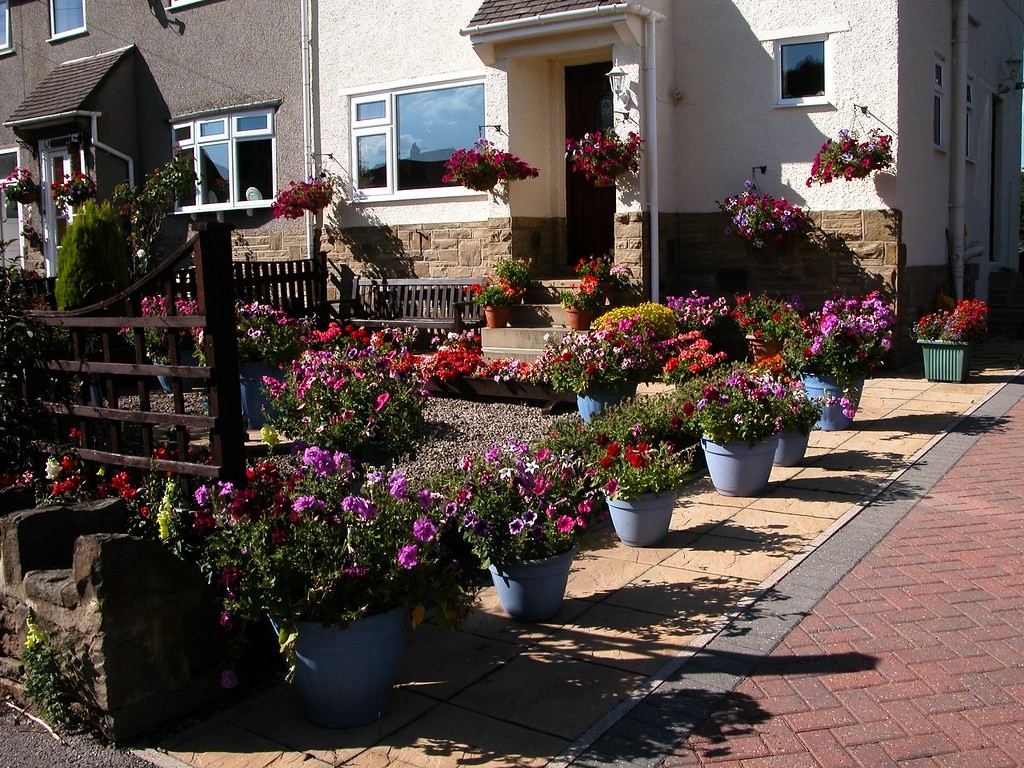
<box><xmin>998</xmin><ymin>53</ymin><xmax>1023</xmax><ymax>95</ymax></box>
<box><xmin>604</xmin><ymin>58</ymin><xmax>629</xmax><ymax>101</ymax></box>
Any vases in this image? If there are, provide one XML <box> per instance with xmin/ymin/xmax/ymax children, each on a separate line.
<box><xmin>153</xmin><ymin>350</ymin><xmax>197</xmax><ymax>393</ymax></box>
<box><xmin>487</xmin><ymin>540</ymin><xmax>578</xmax><ymax>617</ymax></box>
<box><xmin>564</xmin><ymin>305</ymin><xmax>591</xmax><ymax>330</ymax></box>
<box><xmin>578</xmin><ymin>368</ymin><xmax>641</xmax><ymax>427</ymax></box>
<box><xmin>30</xmin><ymin>237</ymin><xmax>39</xmax><ymax>247</ymax></box>
<box><xmin>483</xmin><ymin>305</ymin><xmax>509</xmax><ymax>328</ymax></box>
<box><xmin>67</xmin><ymin>193</ymin><xmax>84</xmax><ymax>205</ymax></box>
<box><xmin>752</xmin><ymin>226</ymin><xmax>787</xmax><ymax>257</ymax></box>
<box><xmin>269</xmin><ymin>604</ymin><xmax>407</xmax><ymax>714</ymax></box>
<box><xmin>509</xmin><ymin>283</ymin><xmax>525</xmax><ymax>304</ymax></box>
<box><xmin>239</xmin><ymin>354</ymin><xmax>287</xmax><ymax>430</ymax></box>
<box><xmin>604</xmin><ymin>485</ymin><xmax>679</xmax><ymax>546</ymax></box>
<box><xmin>701</xmin><ymin>431</ymin><xmax>781</xmax><ymax>496</ymax></box>
<box><xmin>744</xmin><ymin>333</ymin><xmax>781</xmax><ymax>363</ymax></box>
<box><xmin>14</xmin><ymin>192</ymin><xmax>36</xmax><ymax>205</ymax></box>
<box><xmin>916</xmin><ymin>339</ymin><xmax>971</xmax><ymax>382</ymax></box>
<box><xmin>800</xmin><ymin>368</ymin><xmax>868</xmax><ymax>434</ymax></box>
<box><xmin>598</xmin><ymin>280</ymin><xmax>608</xmax><ymax>306</ymax></box>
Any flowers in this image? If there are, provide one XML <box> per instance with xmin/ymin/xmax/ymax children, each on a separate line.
<box><xmin>0</xmin><ymin>129</ymin><xmax>990</xmax><ymax>683</ymax></box>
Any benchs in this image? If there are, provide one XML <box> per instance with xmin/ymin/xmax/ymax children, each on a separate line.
<box><xmin>326</xmin><ymin>275</ymin><xmax>488</xmax><ymax>337</ymax></box>
